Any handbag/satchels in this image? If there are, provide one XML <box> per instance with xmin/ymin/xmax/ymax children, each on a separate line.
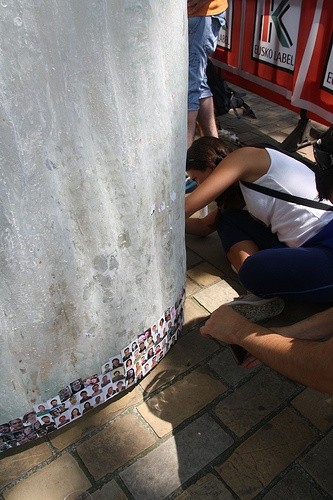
<box><xmin>235</xmin><ymin>124</ymin><xmax>333</xmax><ymax>212</ymax></box>
<box><xmin>205</xmin><ymin>58</ymin><xmax>247</xmax><ymax>116</ymax></box>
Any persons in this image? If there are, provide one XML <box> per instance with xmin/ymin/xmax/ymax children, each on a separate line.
<box><xmin>200</xmin><ymin>124</ymin><xmax>332</xmax><ymax>393</ymax></box>
<box><xmin>185</xmin><ymin>0</ymin><xmax>228</xmax><ymax>193</ymax></box>
<box><xmin>185</xmin><ymin>135</ymin><xmax>333</xmax><ymax>323</ymax></box>
<box><xmin>0</xmin><ymin>289</ymin><xmax>186</xmax><ymax>453</ymax></box>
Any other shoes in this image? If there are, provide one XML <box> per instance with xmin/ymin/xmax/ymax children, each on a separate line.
<box><xmin>225</xmin><ymin>294</ymin><xmax>286</xmax><ymax>323</ymax></box>
